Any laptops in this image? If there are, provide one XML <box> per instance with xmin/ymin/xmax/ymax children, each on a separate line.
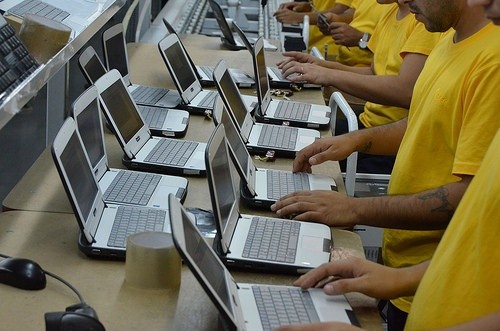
<box><xmin>210</xmin><ymin>94</ymin><xmax>337</xmax><ymax>210</ymax></box>
<box><xmin>50</xmin><ymin>116</ymin><xmax>197</xmax><ymax>262</ymax></box>
<box><xmin>92</xmin><ymin>68</ymin><xmax>208</xmax><ymax>176</ymax></box>
<box><xmin>206</xmin><ymin>122</ymin><xmax>335</xmax><ymax>273</ymax></box>
<box><xmin>79</xmin><ymin>46</ymin><xmax>191</xmax><ymax>138</ymax></box>
<box><xmin>104</xmin><ymin>22</ymin><xmax>184</xmax><ymax>109</ymax></box>
<box><xmin>232</xmin><ymin>20</ymin><xmax>324</xmax><ymax>90</ymax></box>
<box><xmin>159</xmin><ymin>32</ymin><xmax>260</xmax><ymax>115</ymax></box>
<box><xmin>212</xmin><ymin>59</ymin><xmax>321</xmax><ymax>156</ymax></box>
<box><xmin>162</xmin><ymin>17</ymin><xmax>256</xmax><ymax>87</ymax></box>
<box><xmin>167</xmin><ymin>192</ymin><xmax>362</xmax><ymax>331</ymax></box>
<box><xmin>72</xmin><ymin>84</ymin><xmax>189</xmax><ymax>207</ymax></box>
<box><xmin>208</xmin><ymin>0</ymin><xmax>260</xmax><ymax>50</ymax></box>
<box><xmin>252</xmin><ymin>36</ymin><xmax>333</xmax><ymax>127</ymax></box>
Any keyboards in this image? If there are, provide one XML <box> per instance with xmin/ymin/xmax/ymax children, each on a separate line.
<box><xmin>2</xmin><ymin>24</ymin><xmax>41</xmax><ymax>107</ymax></box>
<box><xmin>7</xmin><ymin>0</ymin><xmax>70</xmax><ymax>22</ymax></box>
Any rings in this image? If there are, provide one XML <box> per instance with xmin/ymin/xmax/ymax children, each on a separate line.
<box><xmin>301</xmin><ymin>67</ymin><xmax>304</xmax><ymax>73</ymax></box>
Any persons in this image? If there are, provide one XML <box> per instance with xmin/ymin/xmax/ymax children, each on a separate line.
<box><xmin>272</xmin><ymin>0</ymin><xmax>499</xmax><ymax>330</ymax></box>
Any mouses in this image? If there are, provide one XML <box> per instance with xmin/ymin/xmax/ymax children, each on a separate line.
<box><xmin>0</xmin><ymin>256</ymin><xmax>48</xmax><ymax>291</ymax></box>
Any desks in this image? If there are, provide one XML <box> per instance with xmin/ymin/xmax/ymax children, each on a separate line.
<box><xmin>0</xmin><ymin>0</ymin><xmax>380</xmax><ymax>331</ymax></box>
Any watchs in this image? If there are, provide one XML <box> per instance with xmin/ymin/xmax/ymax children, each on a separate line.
<box><xmin>358</xmin><ymin>31</ymin><xmax>369</xmax><ymax>49</ymax></box>
